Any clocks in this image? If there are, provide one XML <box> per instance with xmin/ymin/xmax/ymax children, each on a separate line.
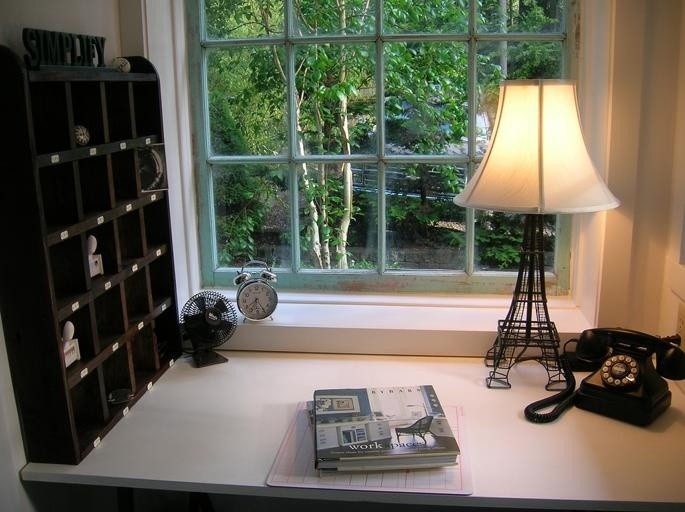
<box><xmin>234</xmin><ymin>260</ymin><xmax>278</xmax><ymax>323</ymax></box>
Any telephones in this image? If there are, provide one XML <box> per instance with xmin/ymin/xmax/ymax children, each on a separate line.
<box><xmin>574</xmin><ymin>328</ymin><xmax>685</xmax><ymax>427</ymax></box>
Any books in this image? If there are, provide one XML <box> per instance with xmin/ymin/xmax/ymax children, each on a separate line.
<box><xmin>306</xmin><ymin>399</ymin><xmax>460</xmax><ymax>472</ymax></box>
<box><xmin>312</xmin><ymin>384</ymin><xmax>462</xmax><ymax>469</ymax></box>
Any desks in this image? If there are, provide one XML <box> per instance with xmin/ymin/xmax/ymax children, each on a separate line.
<box><xmin>20</xmin><ymin>349</ymin><xmax>685</xmax><ymax>512</ymax></box>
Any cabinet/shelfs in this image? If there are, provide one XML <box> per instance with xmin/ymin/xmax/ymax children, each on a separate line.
<box><xmin>0</xmin><ymin>28</ymin><xmax>183</xmax><ymax>465</ymax></box>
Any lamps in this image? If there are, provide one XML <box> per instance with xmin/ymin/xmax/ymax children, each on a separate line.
<box><xmin>453</xmin><ymin>79</ymin><xmax>622</xmax><ymax>391</ymax></box>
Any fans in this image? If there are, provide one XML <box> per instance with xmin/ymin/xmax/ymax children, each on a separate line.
<box><xmin>180</xmin><ymin>291</ymin><xmax>238</xmax><ymax>368</ymax></box>
